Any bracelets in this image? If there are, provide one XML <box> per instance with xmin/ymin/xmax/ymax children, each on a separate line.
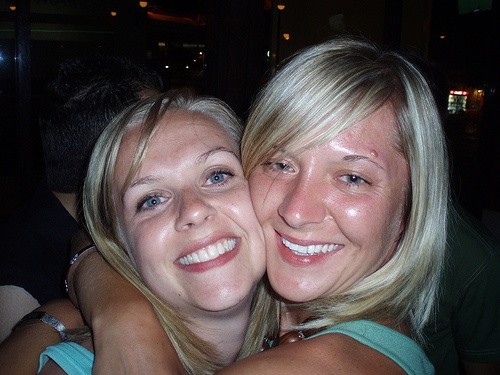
<box><xmin>11</xmin><ymin>310</ymin><xmax>67</xmax><ymax>342</ymax></box>
<box><xmin>64</xmin><ymin>243</ymin><xmax>98</xmax><ymax>309</ymax></box>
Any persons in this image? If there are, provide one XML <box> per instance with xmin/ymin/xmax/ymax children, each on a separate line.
<box><xmin>0</xmin><ymin>32</ymin><xmax>500</xmax><ymax>375</ymax></box>
